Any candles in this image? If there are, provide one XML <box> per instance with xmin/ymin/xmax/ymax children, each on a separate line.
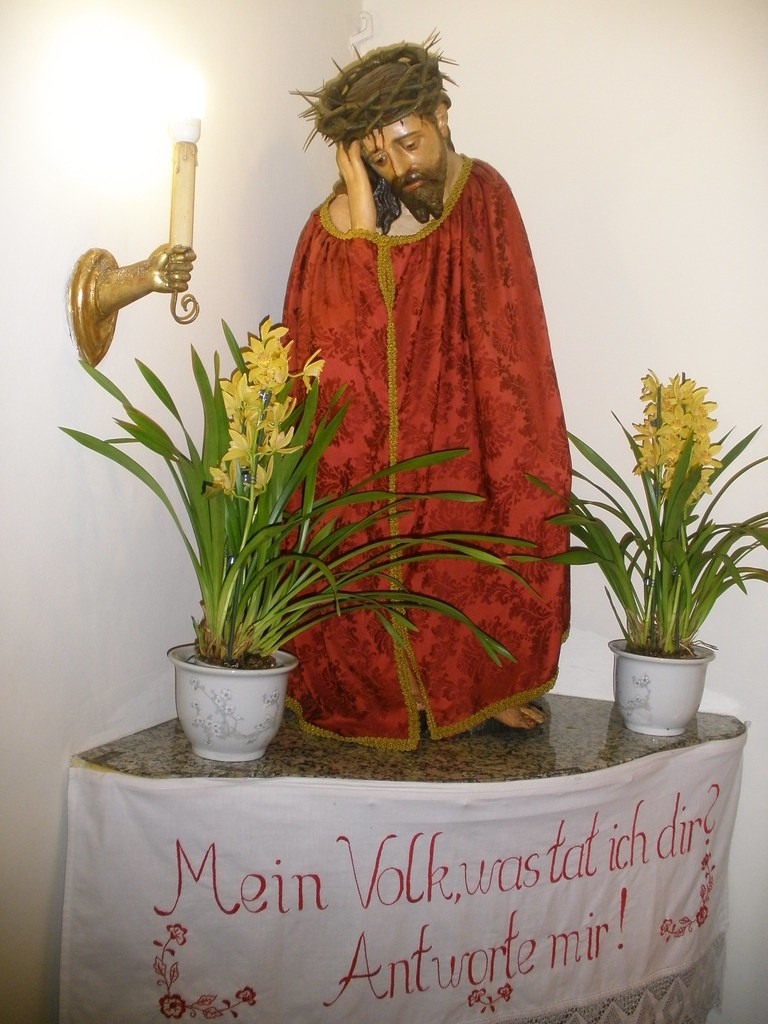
<box><xmin>168</xmin><ymin>112</ymin><xmax>198</xmax><ymax>249</ymax></box>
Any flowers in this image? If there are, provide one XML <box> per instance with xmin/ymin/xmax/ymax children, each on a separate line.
<box><xmin>523</xmin><ymin>367</ymin><xmax>768</xmax><ymax>659</ymax></box>
<box><xmin>61</xmin><ymin>316</ymin><xmax>539</xmax><ymax>668</ymax></box>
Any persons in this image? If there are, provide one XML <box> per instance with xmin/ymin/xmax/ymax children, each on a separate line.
<box><xmin>275</xmin><ymin>25</ymin><xmax>570</xmax><ymax>752</ymax></box>
<box><xmin>97</xmin><ymin>242</ymin><xmax>196</xmax><ymax>314</ymax></box>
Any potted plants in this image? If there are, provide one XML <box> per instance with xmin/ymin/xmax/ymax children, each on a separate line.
<box><xmin>166</xmin><ymin>646</ymin><xmax>298</xmax><ymax>763</ymax></box>
<box><xmin>607</xmin><ymin>639</ymin><xmax>716</xmax><ymax>737</ymax></box>
